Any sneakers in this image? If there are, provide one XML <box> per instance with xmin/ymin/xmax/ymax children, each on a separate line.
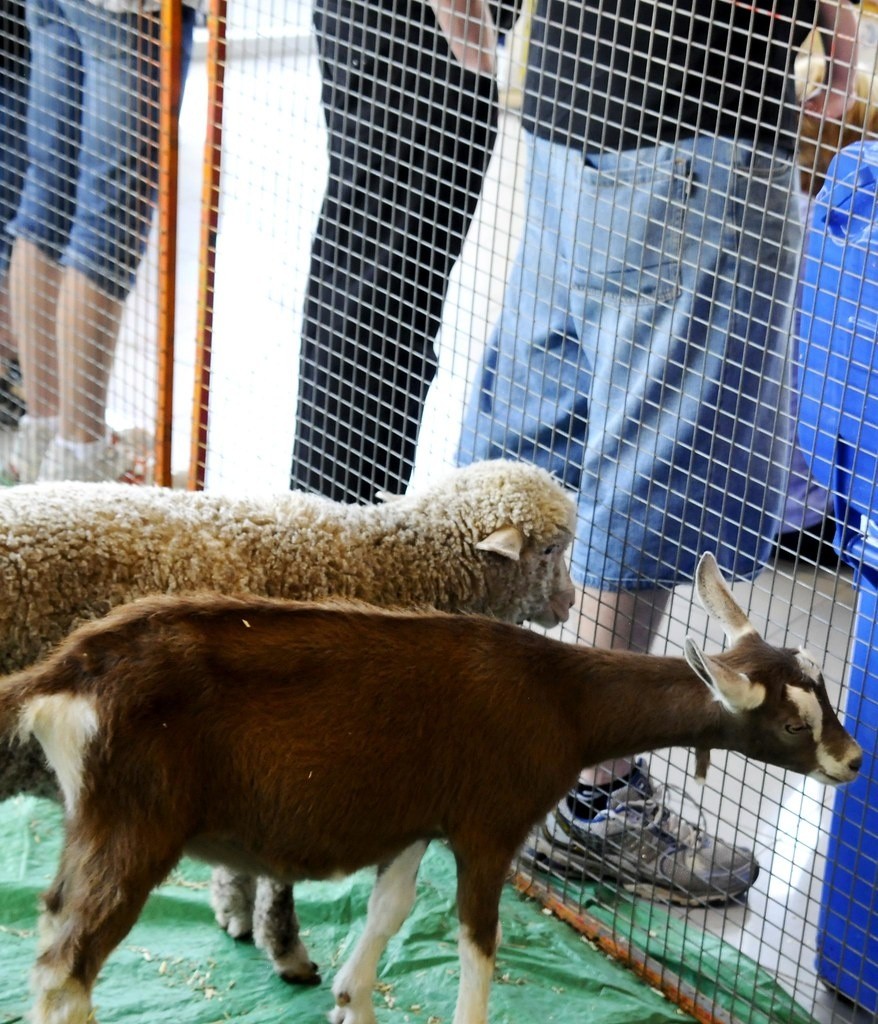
<box><xmin>524</xmin><ymin>757</ymin><xmax>760</xmax><ymax>906</ymax></box>
<box><xmin>0</xmin><ymin>357</ymin><xmax>190</xmax><ymax>491</ymax></box>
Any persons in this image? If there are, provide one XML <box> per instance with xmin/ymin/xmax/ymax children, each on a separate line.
<box><xmin>453</xmin><ymin>2</ymin><xmax>809</xmax><ymax>912</ymax></box>
<box><xmin>290</xmin><ymin>0</ymin><xmax>527</xmax><ymax>509</ymax></box>
<box><xmin>2</xmin><ymin>0</ymin><xmax>36</xmax><ymax>428</ymax></box>
<box><xmin>8</xmin><ymin>3</ymin><xmax>203</xmax><ymax>490</ymax></box>
<box><xmin>800</xmin><ymin>1</ymin><xmax>860</xmax><ymax>121</ymax></box>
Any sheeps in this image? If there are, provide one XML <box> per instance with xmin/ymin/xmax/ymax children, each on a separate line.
<box><xmin>0</xmin><ymin>457</ymin><xmax>576</xmax><ymax>987</ymax></box>
<box><xmin>0</xmin><ymin>549</ymin><xmax>864</xmax><ymax>1024</ymax></box>
